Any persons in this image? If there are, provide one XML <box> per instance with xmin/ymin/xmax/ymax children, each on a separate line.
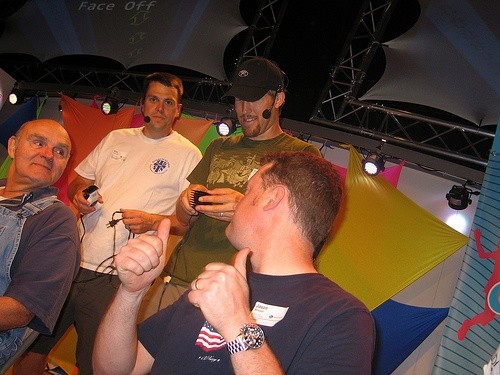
<box><xmin>92</xmin><ymin>150</ymin><xmax>376</xmax><ymax>375</ymax></box>
<box><xmin>13</xmin><ymin>72</ymin><xmax>203</xmax><ymax>375</ymax></box>
<box><xmin>0</xmin><ymin>119</ymin><xmax>81</xmax><ymax>369</ymax></box>
<box><xmin>137</xmin><ymin>58</ymin><xmax>323</xmax><ymax>324</ymax></box>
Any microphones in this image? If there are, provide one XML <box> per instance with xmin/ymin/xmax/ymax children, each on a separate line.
<box><xmin>262</xmin><ymin>93</ymin><xmax>279</xmax><ymax>119</ymax></box>
<box><xmin>141</xmin><ymin>107</ymin><xmax>151</xmax><ymax>123</ymax></box>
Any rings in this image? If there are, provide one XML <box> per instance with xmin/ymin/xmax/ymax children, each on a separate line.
<box><xmin>195</xmin><ymin>278</ymin><xmax>202</xmax><ymax>290</ymax></box>
<box><xmin>129</xmin><ymin>225</ymin><xmax>130</xmax><ymax>229</ymax></box>
<box><xmin>220</xmin><ymin>213</ymin><xmax>223</xmax><ymax>217</ymax></box>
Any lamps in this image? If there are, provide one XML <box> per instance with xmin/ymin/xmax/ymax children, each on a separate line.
<box><xmin>213</xmin><ymin>115</ymin><xmax>240</xmax><ymax>137</ymax></box>
<box><xmin>446</xmin><ymin>180</ymin><xmax>480</xmax><ymax>210</ymax></box>
<box><xmin>361</xmin><ymin>151</ymin><xmax>386</xmax><ymax>176</ymax></box>
<box><xmin>8</xmin><ymin>88</ymin><xmax>27</xmax><ymax>105</ymax></box>
<box><xmin>101</xmin><ymin>96</ymin><xmax>128</xmax><ymax>115</ymax></box>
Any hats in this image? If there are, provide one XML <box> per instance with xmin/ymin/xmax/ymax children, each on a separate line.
<box><xmin>221</xmin><ymin>57</ymin><xmax>284</xmax><ymax>102</ymax></box>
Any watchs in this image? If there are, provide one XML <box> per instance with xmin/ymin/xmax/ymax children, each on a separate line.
<box><xmin>228</xmin><ymin>323</ymin><xmax>264</xmax><ymax>355</ymax></box>
